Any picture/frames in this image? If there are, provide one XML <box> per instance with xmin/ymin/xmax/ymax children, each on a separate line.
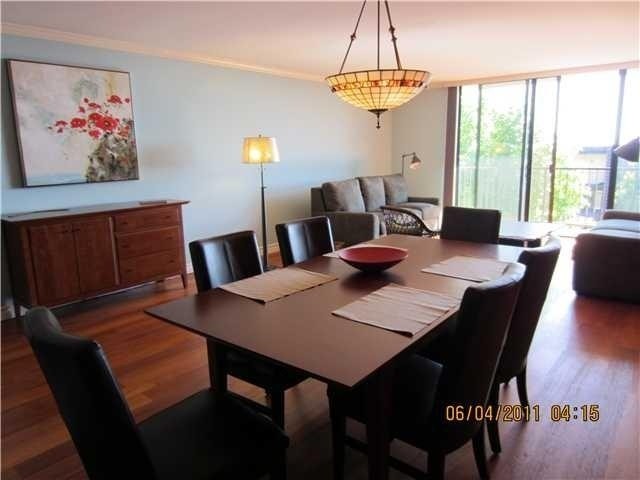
<box><xmin>6</xmin><ymin>59</ymin><xmax>139</xmax><ymax>187</ymax></box>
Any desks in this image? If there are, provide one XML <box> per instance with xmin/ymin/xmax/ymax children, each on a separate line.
<box><xmin>143</xmin><ymin>235</ymin><xmax>532</xmax><ymax>480</ymax></box>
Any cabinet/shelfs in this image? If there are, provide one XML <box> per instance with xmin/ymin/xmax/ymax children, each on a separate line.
<box><xmin>0</xmin><ymin>192</ymin><xmax>190</xmax><ymax>308</ymax></box>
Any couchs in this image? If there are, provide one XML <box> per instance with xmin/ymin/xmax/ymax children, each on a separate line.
<box><xmin>310</xmin><ymin>174</ymin><xmax>443</xmax><ymax>244</ymax></box>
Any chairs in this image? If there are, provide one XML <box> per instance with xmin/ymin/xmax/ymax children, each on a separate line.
<box><xmin>380</xmin><ymin>205</ymin><xmax>440</xmax><ymax>238</ymax></box>
<box><xmin>22</xmin><ymin>305</ymin><xmax>288</xmax><ymax>479</ymax></box>
<box><xmin>189</xmin><ymin>230</ymin><xmax>309</xmax><ymax>427</ymax></box>
<box><xmin>277</xmin><ymin>216</ymin><xmax>335</xmax><ymax>265</ymax></box>
<box><xmin>490</xmin><ymin>232</ymin><xmax>562</xmax><ymax>454</ymax></box>
<box><xmin>570</xmin><ymin>209</ymin><xmax>640</xmax><ymax>300</ymax></box>
<box><xmin>327</xmin><ymin>260</ymin><xmax>528</xmax><ymax>480</ymax></box>
<box><xmin>440</xmin><ymin>206</ymin><xmax>501</xmax><ymax>242</ymax></box>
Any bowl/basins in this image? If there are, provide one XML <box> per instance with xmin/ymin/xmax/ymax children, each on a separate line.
<box><xmin>338</xmin><ymin>245</ymin><xmax>408</xmax><ymax>275</ymax></box>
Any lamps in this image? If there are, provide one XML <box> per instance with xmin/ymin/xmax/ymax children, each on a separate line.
<box><xmin>401</xmin><ymin>152</ymin><xmax>422</xmax><ymax>176</ymax></box>
<box><xmin>243</xmin><ymin>135</ymin><xmax>281</xmax><ymax>271</ymax></box>
<box><xmin>325</xmin><ymin>0</ymin><xmax>432</xmax><ymax>131</ymax></box>
<box><xmin>614</xmin><ymin>137</ymin><xmax>640</xmax><ymax>163</ymax></box>
